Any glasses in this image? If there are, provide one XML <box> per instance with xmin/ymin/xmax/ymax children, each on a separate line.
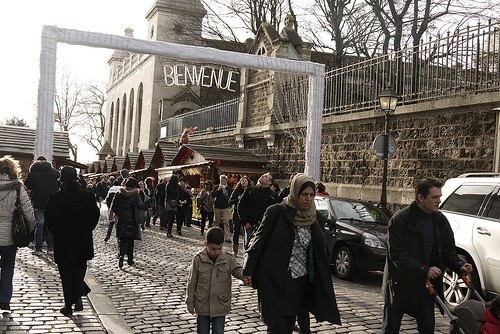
<box><xmin>104</xmin><ymin>176</ymin><xmax>108</xmax><ymax>178</ymax></box>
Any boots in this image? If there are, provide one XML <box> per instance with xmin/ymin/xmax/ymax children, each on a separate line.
<box><xmin>104</xmin><ymin>221</ymin><xmax>114</xmax><ymax>243</ymax></box>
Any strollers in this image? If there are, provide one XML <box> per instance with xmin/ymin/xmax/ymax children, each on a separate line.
<box><xmin>425</xmin><ymin>273</ymin><xmax>487</xmax><ymax>334</ymax></box>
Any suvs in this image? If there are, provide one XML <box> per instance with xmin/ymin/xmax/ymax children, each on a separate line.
<box><xmin>431</xmin><ymin>171</ymin><xmax>500</xmax><ymax>313</ymax></box>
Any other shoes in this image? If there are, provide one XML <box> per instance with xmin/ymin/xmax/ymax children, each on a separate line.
<box><xmin>167</xmin><ymin>232</ymin><xmax>173</xmax><ymax>237</ymax></box>
<box><xmin>118</xmin><ymin>255</ymin><xmax>124</xmax><ymax>268</ymax></box>
<box><xmin>60</xmin><ymin>307</ymin><xmax>72</xmax><ymax>316</ymax></box>
<box><xmin>0</xmin><ymin>303</ymin><xmax>10</xmax><ymax>310</ymax></box>
<box><xmin>177</xmin><ymin>231</ymin><xmax>182</xmax><ymax>236</ymax></box>
<box><xmin>74</xmin><ymin>304</ymin><xmax>84</xmax><ymax>312</ymax></box>
<box><xmin>225</xmin><ymin>239</ymin><xmax>232</xmax><ymax>243</ymax></box>
<box><xmin>127</xmin><ymin>261</ymin><xmax>135</xmax><ymax>266</ymax></box>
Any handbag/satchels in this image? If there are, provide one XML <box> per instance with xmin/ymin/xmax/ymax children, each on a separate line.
<box><xmin>11</xmin><ymin>207</ymin><xmax>29</xmax><ymax>247</ymax></box>
<box><xmin>134</xmin><ymin>207</ymin><xmax>144</xmax><ymax>224</ymax></box>
<box><xmin>169</xmin><ymin>200</ymin><xmax>178</xmax><ymax>209</ymax></box>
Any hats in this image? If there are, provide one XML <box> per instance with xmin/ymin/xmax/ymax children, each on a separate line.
<box><xmin>204</xmin><ymin>180</ymin><xmax>213</xmax><ymax>185</ymax></box>
<box><xmin>57</xmin><ymin>166</ymin><xmax>80</xmax><ymax>181</ymax></box>
<box><xmin>107</xmin><ymin>182</ymin><xmax>111</xmax><ymax>185</ymax></box>
<box><xmin>146</xmin><ymin>178</ymin><xmax>153</xmax><ymax>182</ymax></box>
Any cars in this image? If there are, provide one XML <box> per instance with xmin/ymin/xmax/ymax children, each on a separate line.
<box><xmin>314</xmin><ymin>195</ymin><xmax>395</xmax><ymax>279</ymax></box>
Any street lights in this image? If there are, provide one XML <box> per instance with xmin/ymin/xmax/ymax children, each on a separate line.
<box><xmin>376</xmin><ymin>84</ymin><xmax>399</xmax><ymax>211</ymax></box>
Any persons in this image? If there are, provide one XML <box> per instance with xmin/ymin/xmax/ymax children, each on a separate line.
<box><xmin>97</xmin><ymin>169</ymin><xmax>231</xmax><ymax>243</ymax></box>
<box><xmin>272</xmin><ymin>173</ymin><xmax>298</xmax><ymax>201</ymax></box>
<box><xmin>243</xmin><ymin>173</ymin><xmax>342</xmax><ymax>334</ymax></box>
<box><xmin>315</xmin><ymin>183</ymin><xmax>329</xmax><ymax>196</ymax></box>
<box><xmin>24</xmin><ymin>155</ymin><xmax>60</xmax><ymax>255</ymax></box>
<box><xmin>185</xmin><ymin>227</ymin><xmax>249</xmax><ymax>334</ymax></box>
<box><xmin>481</xmin><ymin>295</ymin><xmax>500</xmax><ymax>334</ymax></box>
<box><xmin>44</xmin><ymin>165</ymin><xmax>100</xmax><ymax>316</ymax></box>
<box><xmin>227</xmin><ymin>176</ymin><xmax>254</xmax><ymax>257</ymax></box>
<box><xmin>0</xmin><ymin>155</ymin><xmax>36</xmax><ymax>310</ymax></box>
<box><xmin>382</xmin><ymin>177</ymin><xmax>472</xmax><ymax>334</ymax></box>
<box><xmin>237</xmin><ymin>172</ymin><xmax>280</xmax><ymax>247</ymax></box>
<box><xmin>111</xmin><ymin>176</ymin><xmax>144</xmax><ymax>267</ymax></box>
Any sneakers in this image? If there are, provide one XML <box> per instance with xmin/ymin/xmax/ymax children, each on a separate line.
<box><xmin>30</xmin><ymin>249</ymin><xmax>42</xmax><ymax>255</ymax></box>
<box><xmin>45</xmin><ymin>250</ymin><xmax>53</xmax><ymax>255</ymax></box>
